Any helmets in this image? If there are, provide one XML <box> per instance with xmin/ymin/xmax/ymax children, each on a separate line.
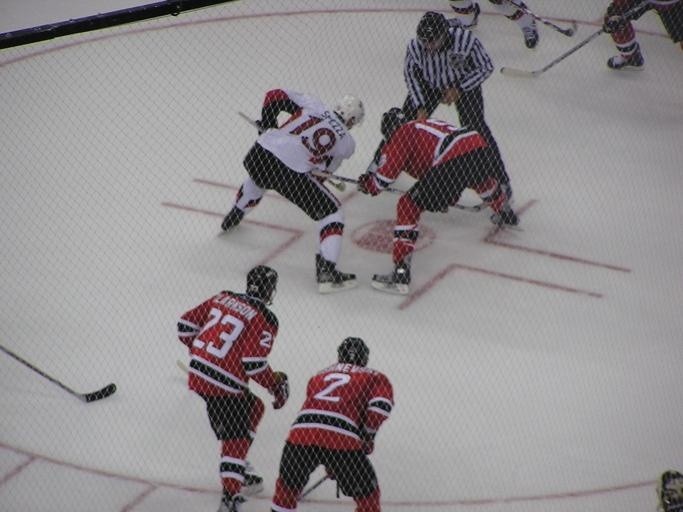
<box><xmin>381</xmin><ymin>107</ymin><xmax>408</xmax><ymax>142</ymax></box>
<box><xmin>333</xmin><ymin>93</ymin><xmax>365</xmax><ymax>130</ymax></box>
<box><xmin>338</xmin><ymin>337</ymin><xmax>368</xmax><ymax>367</ymax></box>
<box><xmin>417</xmin><ymin>10</ymin><xmax>450</xmax><ymax>40</ymax></box>
<box><xmin>246</xmin><ymin>266</ymin><xmax>278</xmax><ymax>306</ymax></box>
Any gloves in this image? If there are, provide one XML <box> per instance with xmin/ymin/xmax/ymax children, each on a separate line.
<box><xmin>358</xmin><ymin>174</ymin><xmax>372</xmax><ymax>195</ymax></box>
<box><xmin>270</xmin><ymin>372</ymin><xmax>289</xmax><ymax>408</ymax></box>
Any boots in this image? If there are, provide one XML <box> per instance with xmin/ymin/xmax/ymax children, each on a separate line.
<box><xmin>447</xmin><ymin>3</ymin><xmax>479</xmax><ymax>31</ymax></box>
<box><xmin>222</xmin><ymin>473</ymin><xmax>263</xmax><ymax>512</ymax></box>
<box><xmin>371</xmin><ymin>250</ymin><xmax>413</xmax><ymax>285</ymax></box>
<box><xmin>522</xmin><ymin>18</ymin><xmax>539</xmax><ymax>48</ymax></box>
<box><xmin>220</xmin><ymin>205</ymin><xmax>244</xmax><ymax>232</ymax></box>
<box><xmin>606</xmin><ymin>43</ymin><xmax>644</xmax><ymax>69</ymax></box>
<box><xmin>315</xmin><ymin>253</ymin><xmax>356</xmax><ymax>284</ymax></box>
<box><xmin>488</xmin><ymin>182</ymin><xmax>519</xmax><ymax>228</ymax></box>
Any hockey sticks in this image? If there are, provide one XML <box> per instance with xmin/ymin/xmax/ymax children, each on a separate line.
<box><xmin>499</xmin><ymin>1</ymin><xmax>649</xmax><ymax>80</ymax></box>
<box><xmin>238</xmin><ymin>110</ymin><xmax>347</xmax><ymax>193</ymax></box>
<box><xmin>505</xmin><ymin>0</ymin><xmax>578</xmax><ymax>38</ymax></box>
<box><xmin>0</xmin><ymin>344</ymin><xmax>116</xmax><ymax>403</ymax></box>
<box><xmin>310</xmin><ymin>169</ymin><xmax>492</xmax><ymax>213</ymax></box>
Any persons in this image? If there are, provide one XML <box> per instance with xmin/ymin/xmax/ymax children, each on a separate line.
<box><xmin>653</xmin><ymin>468</ymin><xmax>681</xmax><ymax>511</ymax></box>
<box><xmin>444</xmin><ymin>2</ymin><xmax>539</xmax><ymax>48</ymax></box>
<box><xmin>219</xmin><ymin>87</ymin><xmax>363</xmax><ymax>292</ymax></box>
<box><xmin>177</xmin><ymin>264</ymin><xmax>288</xmax><ymax>512</ymax></box>
<box><xmin>356</xmin><ymin>11</ymin><xmax>513</xmax><ymax>202</ymax></box>
<box><xmin>603</xmin><ymin>1</ymin><xmax>682</xmax><ymax>72</ymax></box>
<box><xmin>270</xmin><ymin>336</ymin><xmax>394</xmax><ymax>512</ymax></box>
<box><xmin>358</xmin><ymin>107</ymin><xmax>523</xmax><ymax>295</ymax></box>
